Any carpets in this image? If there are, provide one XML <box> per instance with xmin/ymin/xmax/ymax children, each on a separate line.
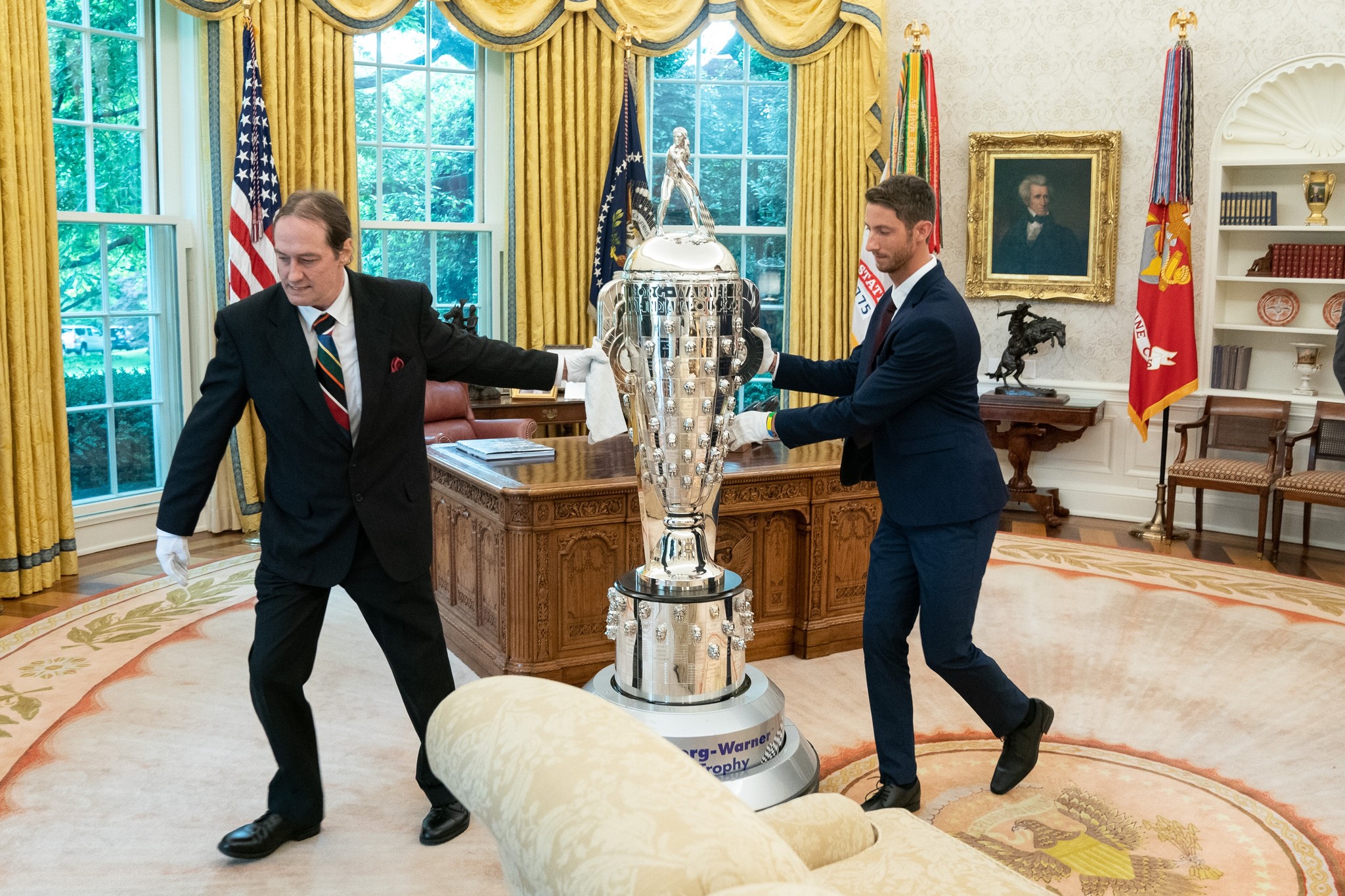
<box><xmin>2</xmin><ymin>525</ymin><xmax>1345</xmax><ymax>896</ymax></box>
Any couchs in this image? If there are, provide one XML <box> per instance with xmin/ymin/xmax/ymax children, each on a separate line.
<box><xmin>425</xmin><ymin>379</ymin><xmax>535</xmax><ymax>445</ymax></box>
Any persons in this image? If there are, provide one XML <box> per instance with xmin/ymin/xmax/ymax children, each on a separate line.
<box><xmin>728</xmin><ymin>173</ymin><xmax>1054</xmax><ymax>808</ymax></box>
<box><xmin>464</xmin><ymin>304</ymin><xmax>479</xmax><ymax>334</ymax></box>
<box><xmin>156</xmin><ymin>191</ymin><xmax>608</xmax><ymax>862</ymax></box>
<box><xmin>1247</xmin><ymin>244</ymin><xmax>1272</xmax><ymax>272</ymax></box>
<box><xmin>1332</xmin><ymin>301</ymin><xmax>1345</xmax><ymax>395</ymax></box>
<box><xmin>997</xmin><ymin>302</ymin><xmax>1046</xmax><ymax>351</ymax></box>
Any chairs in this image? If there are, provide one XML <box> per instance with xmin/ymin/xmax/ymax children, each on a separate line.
<box><xmin>1164</xmin><ymin>394</ymin><xmax>1290</xmax><ymax>559</ymax></box>
<box><xmin>1271</xmin><ymin>402</ymin><xmax>1345</xmax><ymax>563</ymax></box>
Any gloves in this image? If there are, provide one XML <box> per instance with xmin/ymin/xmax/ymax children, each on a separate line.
<box><xmin>564</xmin><ymin>347</ymin><xmax>610</xmax><ymax>383</ymax></box>
<box><xmin>727</xmin><ymin>411</ymin><xmax>778</xmax><ymax>451</ymax></box>
<box><xmin>739</xmin><ymin>326</ymin><xmax>775</xmax><ymax>374</ymax></box>
<box><xmin>155</xmin><ymin>529</ymin><xmax>191</xmax><ymax>587</ymax></box>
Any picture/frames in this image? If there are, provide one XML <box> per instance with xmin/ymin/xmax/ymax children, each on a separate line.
<box><xmin>964</xmin><ymin>129</ymin><xmax>1122</xmax><ymax>305</ymax></box>
<box><xmin>511</xmin><ymin>384</ymin><xmax>559</xmax><ymax>401</ymax></box>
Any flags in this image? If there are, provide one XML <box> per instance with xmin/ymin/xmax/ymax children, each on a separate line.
<box><xmin>227</xmin><ymin>25</ymin><xmax>281</xmax><ymax>304</ymax></box>
<box><xmin>848</xmin><ymin>50</ymin><xmax>944</xmax><ymax>347</ymax></box>
<box><xmin>590</xmin><ymin>58</ymin><xmax>656</xmax><ymax>332</ymax></box>
<box><xmin>1126</xmin><ymin>48</ymin><xmax>1200</xmax><ymax>441</ymax></box>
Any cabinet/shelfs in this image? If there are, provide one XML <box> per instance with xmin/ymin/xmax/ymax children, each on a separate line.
<box><xmin>1198</xmin><ymin>54</ymin><xmax>1345</xmax><ymax>405</ymax></box>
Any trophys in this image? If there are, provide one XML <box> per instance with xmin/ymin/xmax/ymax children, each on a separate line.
<box><xmin>1302</xmin><ymin>170</ymin><xmax>1336</xmax><ymax>225</ymax></box>
<box><xmin>1290</xmin><ymin>343</ymin><xmax>1326</xmax><ymax>396</ymax></box>
<box><xmin>580</xmin><ymin>126</ymin><xmax>819</xmax><ymax>813</ymax></box>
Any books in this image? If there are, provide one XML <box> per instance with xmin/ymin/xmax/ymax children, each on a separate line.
<box><xmin>1210</xmin><ymin>346</ymin><xmax>1252</xmax><ymax>390</ymax></box>
<box><xmin>456</xmin><ymin>436</ymin><xmax>556</xmax><ymax>461</ymax></box>
<box><xmin>1271</xmin><ymin>242</ymin><xmax>1345</xmax><ymax>280</ymax></box>
<box><xmin>1220</xmin><ymin>191</ymin><xmax>1278</xmax><ymax>227</ymax></box>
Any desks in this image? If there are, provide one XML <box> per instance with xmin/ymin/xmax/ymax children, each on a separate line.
<box><xmin>980</xmin><ymin>392</ymin><xmax>1107</xmax><ymax>526</ymax></box>
<box><xmin>470</xmin><ymin>393</ymin><xmax>586</xmax><ymax>424</ymax></box>
<box><xmin>437</xmin><ymin>432</ymin><xmax>883</xmax><ymax>685</ymax></box>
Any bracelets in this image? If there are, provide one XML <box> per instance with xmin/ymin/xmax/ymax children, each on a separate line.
<box><xmin>765</xmin><ymin>412</ymin><xmax>777</xmax><ymax>441</ymax></box>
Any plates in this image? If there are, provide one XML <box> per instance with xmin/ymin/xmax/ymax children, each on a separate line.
<box><xmin>1257</xmin><ymin>288</ymin><xmax>1300</xmax><ymax>326</ymax></box>
<box><xmin>1323</xmin><ymin>291</ymin><xmax>1345</xmax><ymax>329</ymax></box>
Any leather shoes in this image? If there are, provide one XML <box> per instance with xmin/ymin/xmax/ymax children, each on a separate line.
<box><xmin>419</xmin><ymin>802</ymin><xmax>471</xmax><ymax>846</ymax></box>
<box><xmin>859</xmin><ymin>777</ymin><xmax>920</xmax><ymax>812</ymax></box>
<box><xmin>218</xmin><ymin>809</ymin><xmax>321</xmax><ymax>859</ymax></box>
<box><xmin>989</xmin><ymin>698</ymin><xmax>1054</xmax><ymax>794</ymax></box>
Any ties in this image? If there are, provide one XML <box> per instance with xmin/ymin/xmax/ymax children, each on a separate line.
<box><xmin>851</xmin><ymin>297</ymin><xmax>898</xmax><ymax>449</ymax></box>
<box><xmin>312</xmin><ymin>313</ymin><xmax>355</xmax><ymax>446</ymax></box>
